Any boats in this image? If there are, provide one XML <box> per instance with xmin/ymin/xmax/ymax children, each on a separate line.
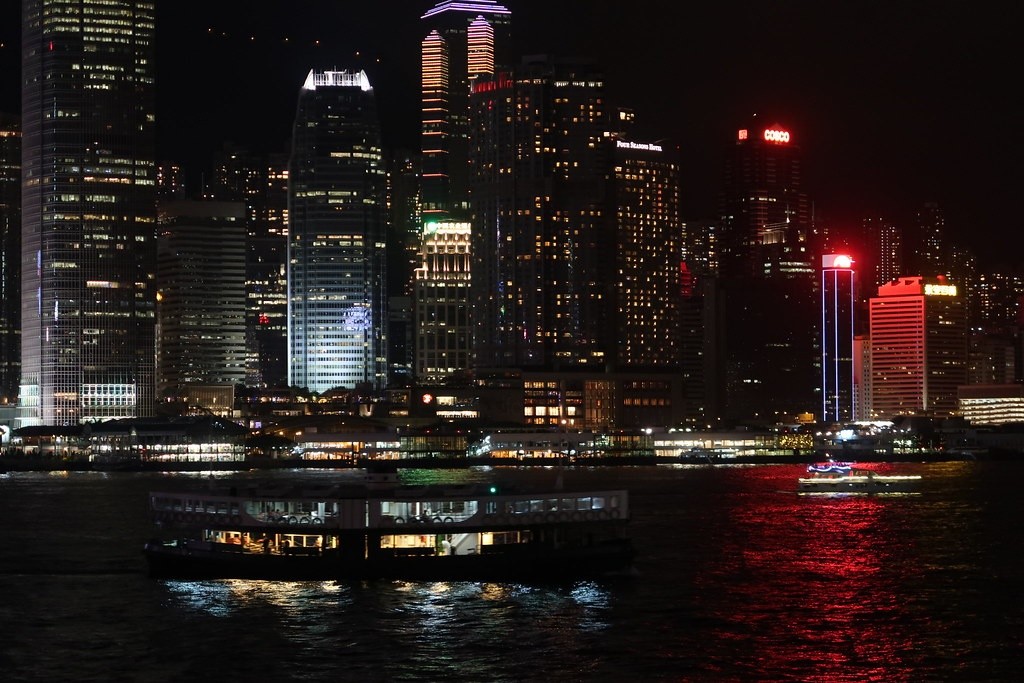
<box><xmin>143</xmin><ymin>489</ymin><xmax>640</xmax><ymax>580</ymax></box>
<box><xmin>799</xmin><ymin>465</ymin><xmax>923</xmax><ymax>498</ymax></box>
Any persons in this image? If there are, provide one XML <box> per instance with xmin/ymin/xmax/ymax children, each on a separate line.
<box><xmin>0</xmin><ymin>436</ymin><xmax>133</xmax><ymax>460</ymax></box>
<box><xmin>427</xmin><ymin>441</ymin><xmax>446</xmax><ymax>450</ymax></box>
<box><xmin>261</xmin><ymin>500</ymin><xmax>558</xmax><ymax>554</ymax></box>
